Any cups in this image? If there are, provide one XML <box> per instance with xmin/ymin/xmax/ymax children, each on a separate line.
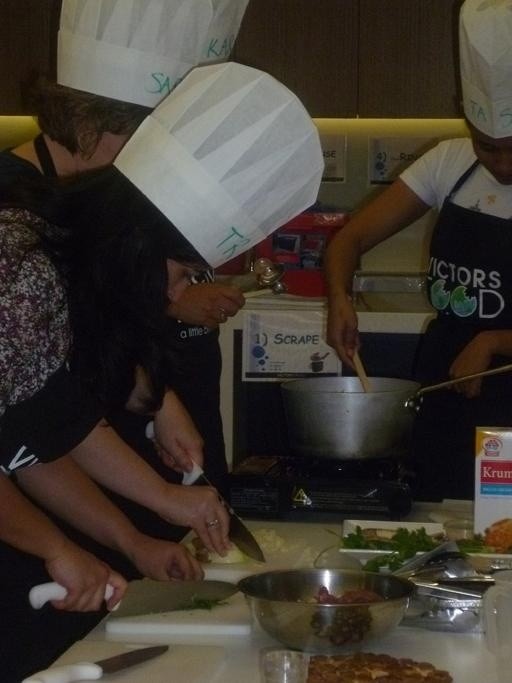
<box><xmin>258</xmin><ymin>648</ymin><xmax>311</xmax><ymax>682</ymax></box>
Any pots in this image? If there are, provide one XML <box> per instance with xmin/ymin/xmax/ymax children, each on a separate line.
<box><xmin>278</xmin><ymin>361</ymin><xmax>511</xmax><ymax>462</ymax></box>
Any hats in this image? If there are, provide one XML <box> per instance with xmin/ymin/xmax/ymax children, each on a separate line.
<box><xmin>57</xmin><ymin>2</ymin><xmax>247</xmax><ymax>110</ymax></box>
<box><xmin>113</xmin><ymin>62</ymin><xmax>325</xmax><ymax>268</ymax></box>
<box><xmin>458</xmin><ymin>0</ymin><xmax>511</xmax><ymax>139</ymax></box>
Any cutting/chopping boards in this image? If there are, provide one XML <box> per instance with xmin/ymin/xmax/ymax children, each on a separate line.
<box><xmin>103</xmin><ymin>569</ymin><xmax>255</xmax><ymax>647</ymax></box>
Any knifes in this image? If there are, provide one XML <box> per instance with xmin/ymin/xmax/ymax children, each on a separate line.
<box><xmin>19</xmin><ymin>643</ymin><xmax>171</xmax><ymax>683</ymax></box>
<box><xmin>25</xmin><ymin>576</ymin><xmax>242</xmax><ymax>621</ymax></box>
<box><xmin>145</xmin><ymin>420</ymin><xmax>266</xmax><ymax>566</ymax></box>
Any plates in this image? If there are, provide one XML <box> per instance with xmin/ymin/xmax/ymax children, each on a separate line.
<box><xmin>338</xmin><ymin>518</ymin><xmax>449</xmax><ymax>557</ymax></box>
<box><xmin>454</xmin><ymin>537</ymin><xmax>512</xmax><ymax>560</ymax></box>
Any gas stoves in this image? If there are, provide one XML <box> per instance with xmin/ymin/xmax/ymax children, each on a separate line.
<box><xmin>224</xmin><ymin>452</ymin><xmax>422</xmax><ymax>521</ymax></box>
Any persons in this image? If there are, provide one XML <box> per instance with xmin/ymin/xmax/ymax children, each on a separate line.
<box><xmin>324</xmin><ymin>114</ymin><xmax>512</xmax><ymax>511</ymax></box>
<box><xmin>1</xmin><ymin>38</ymin><xmax>251</xmax><ymax>682</ymax></box>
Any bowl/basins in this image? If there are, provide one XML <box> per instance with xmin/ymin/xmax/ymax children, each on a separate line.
<box><xmin>235</xmin><ymin>567</ymin><xmax>419</xmax><ymax>656</ymax></box>
